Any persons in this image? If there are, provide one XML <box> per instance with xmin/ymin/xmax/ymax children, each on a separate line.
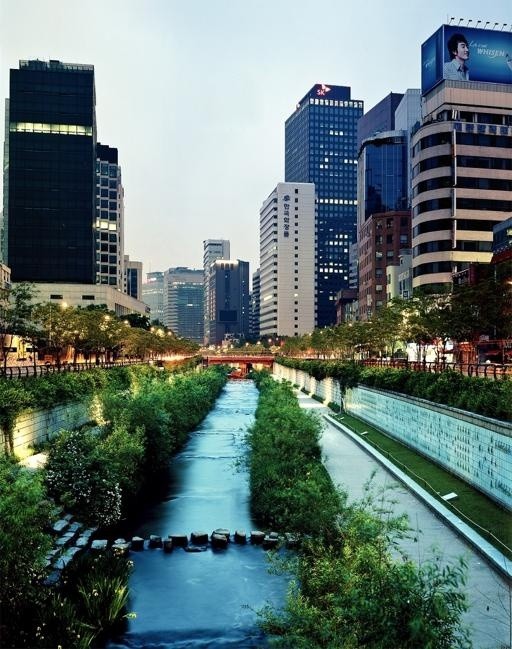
<box><xmin>444</xmin><ymin>33</ymin><xmax>470</xmax><ymax>81</ymax></box>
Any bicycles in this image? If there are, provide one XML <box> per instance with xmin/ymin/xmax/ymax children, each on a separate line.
<box><xmin>432</xmin><ymin>356</ymin><xmax>453</xmax><ymax>376</ymax></box>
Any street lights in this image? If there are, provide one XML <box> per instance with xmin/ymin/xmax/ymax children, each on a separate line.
<box><xmin>96</xmin><ymin>315</ymin><xmax>110</xmax><ymax>365</ymax></box>
<box><xmin>140</xmin><ymin>322</ymin><xmax>410</xmax><ymax>369</ymax></box>
<box><xmin>54</xmin><ymin>302</ymin><xmax>68</xmax><ymax>365</ymax></box>
<box><xmin>116</xmin><ymin>320</ymin><xmax>129</xmax><ymax>363</ymax></box>
